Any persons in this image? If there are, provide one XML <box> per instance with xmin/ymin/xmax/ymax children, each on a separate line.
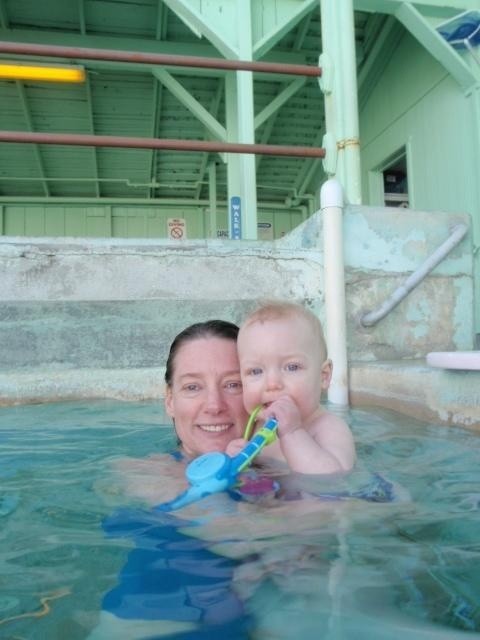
<box><xmin>223</xmin><ymin>302</ymin><xmax>409</xmax><ymax>503</ymax></box>
<box><xmin>83</xmin><ymin>320</ymin><xmax>418</xmax><ymax>639</ymax></box>
